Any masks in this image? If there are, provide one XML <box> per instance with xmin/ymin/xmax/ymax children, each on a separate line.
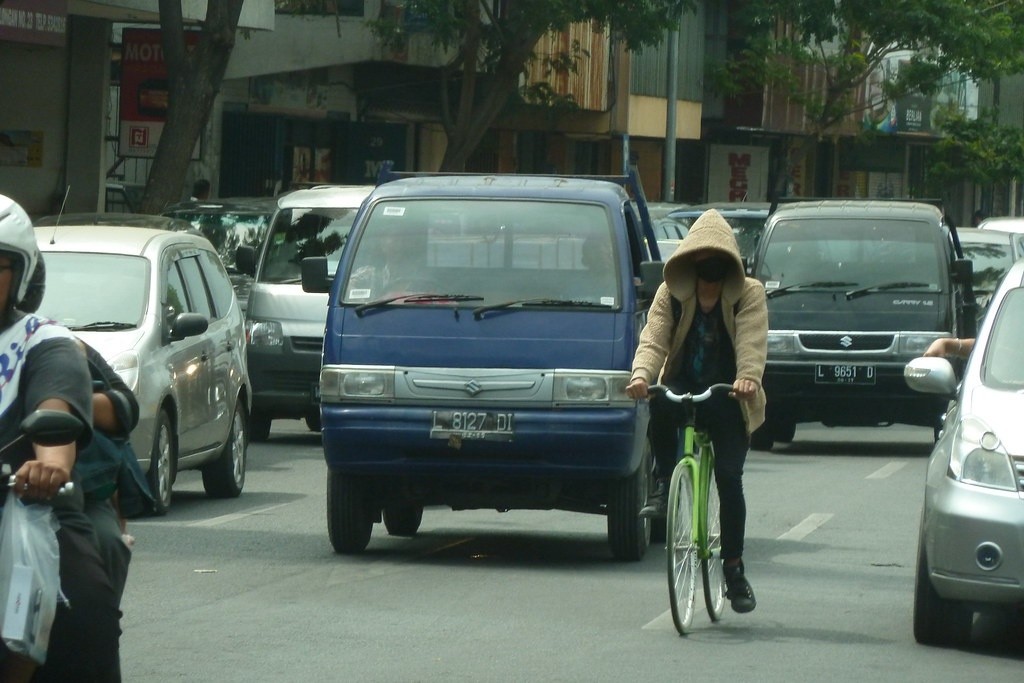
<box><xmin>693</xmin><ymin>257</ymin><xmax>730</xmax><ymax>283</ymax></box>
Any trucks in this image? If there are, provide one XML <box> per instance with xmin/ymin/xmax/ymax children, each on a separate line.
<box><xmin>301</xmin><ymin>161</ymin><xmax>672</xmax><ymax>565</ymax></box>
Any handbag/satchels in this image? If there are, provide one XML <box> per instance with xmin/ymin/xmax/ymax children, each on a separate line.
<box><xmin>0</xmin><ymin>473</ymin><xmax>60</xmax><ymax>666</ymax></box>
<box><xmin>117</xmin><ymin>442</ymin><xmax>157</xmax><ymax>519</ymax></box>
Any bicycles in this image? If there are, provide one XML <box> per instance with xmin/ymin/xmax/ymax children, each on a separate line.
<box><xmin>645</xmin><ymin>382</ymin><xmax>726</xmax><ymax>638</ymax></box>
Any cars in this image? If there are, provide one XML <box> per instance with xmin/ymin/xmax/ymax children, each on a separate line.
<box><xmin>31</xmin><ymin>210</ymin><xmax>254</xmax><ymax>522</ymax></box>
<box><xmin>914</xmin><ymin>260</ymin><xmax>1023</xmax><ymax>649</ymax></box>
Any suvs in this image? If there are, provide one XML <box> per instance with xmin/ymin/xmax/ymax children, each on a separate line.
<box><xmin>152</xmin><ymin>193</ymin><xmax>279</xmax><ymax>314</ymax></box>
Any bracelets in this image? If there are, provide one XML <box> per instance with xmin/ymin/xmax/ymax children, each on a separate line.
<box><xmin>957</xmin><ymin>337</ymin><xmax>963</xmax><ymax>352</ymax></box>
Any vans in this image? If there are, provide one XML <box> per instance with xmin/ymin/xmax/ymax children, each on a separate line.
<box><xmin>242</xmin><ymin>184</ymin><xmax>374</xmax><ymax>442</ymax></box>
<box><xmin>619</xmin><ymin>200</ymin><xmax>1024</xmax><ymax>452</ymax></box>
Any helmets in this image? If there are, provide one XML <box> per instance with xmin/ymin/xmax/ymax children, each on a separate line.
<box><xmin>0</xmin><ymin>193</ymin><xmax>37</xmax><ymax>304</ymax></box>
<box><xmin>16</xmin><ymin>247</ymin><xmax>46</xmax><ymax>314</ymax></box>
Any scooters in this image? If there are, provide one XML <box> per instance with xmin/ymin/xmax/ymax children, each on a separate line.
<box><xmin>1</xmin><ymin>406</ymin><xmax>137</xmax><ymax>682</ymax></box>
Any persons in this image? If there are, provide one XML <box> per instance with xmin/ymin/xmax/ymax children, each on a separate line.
<box><xmin>974</xmin><ymin>209</ymin><xmax>987</xmax><ymax>223</ymax></box>
<box><xmin>0</xmin><ymin>190</ymin><xmax>140</xmax><ymax>683</ymax></box>
<box><xmin>348</xmin><ymin>221</ymin><xmax>432</xmax><ymax>300</ymax></box>
<box><xmin>188</xmin><ymin>179</ymin><xmax>210</xmax><ymax>202</ymax></box>
<box><xmin>624</xmin><ymin>208</ymin><xmax>769</xmax><ymax>614</ymax></box>
<box><xmin>922</xmin><ymin>337</ymin><xmax>977</xmax><ymax>360</ymax></box>
<box><xmin>561</xmin><ymin>232</ymin><xmax>641</xmax><ymax>307</ymax></box>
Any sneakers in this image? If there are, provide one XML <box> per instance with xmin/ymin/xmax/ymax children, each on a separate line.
<box><xmin>638</xmin><ymin>480</ymin><xmax>670</xmax><ymax>518</ymax></box>
<box><xmin>722</xmin><ymin>558</ymin><xmax>756</xmax><ymax>612</ymax></box>
